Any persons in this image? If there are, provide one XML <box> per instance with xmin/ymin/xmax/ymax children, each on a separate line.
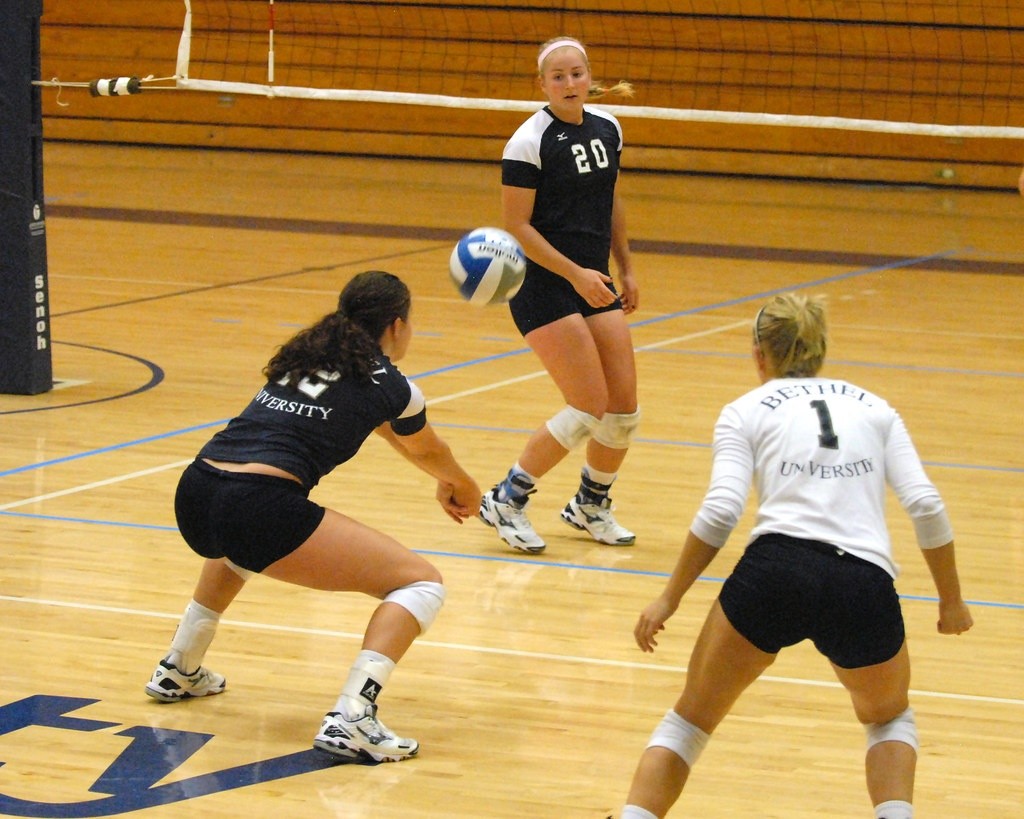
<box><xmin>145</xmin><ymin>271</ymin><xmax>482</xmax><ymax>763</ymax></box>
<box><xmin>475</xmin><ymin>36</ymin><xmax>639</xmax><ymax>555</ymax></box>
<box><xmin>621</xmin><ymin>291</ymin><xmax>973</xmax><ymax>819</ymax></box>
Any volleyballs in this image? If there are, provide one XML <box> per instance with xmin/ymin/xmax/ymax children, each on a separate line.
<box><xmin>447</xmin><ymin>225</ymin><xmax>530</xmax><ymax>308</ymax></box>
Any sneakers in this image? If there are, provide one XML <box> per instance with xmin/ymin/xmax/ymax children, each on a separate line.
<box><xmin>561</xmin><ymin>491</ymin><xmax>637</xmax><ymax>547</ymax></box>
<box><xmin>476</xmin><ymin>486</ymin><xmax>548</xmax><ymax>556</ymax></box>
<box><xmin>311</xmin><ymin>703</ymin><xmax>421</xmax><ymax>766</ymax></box>
<box><xmin>143</xmin><ymin>659</ymin><xmax>228</xmax><ymax>706</ymax></box>
